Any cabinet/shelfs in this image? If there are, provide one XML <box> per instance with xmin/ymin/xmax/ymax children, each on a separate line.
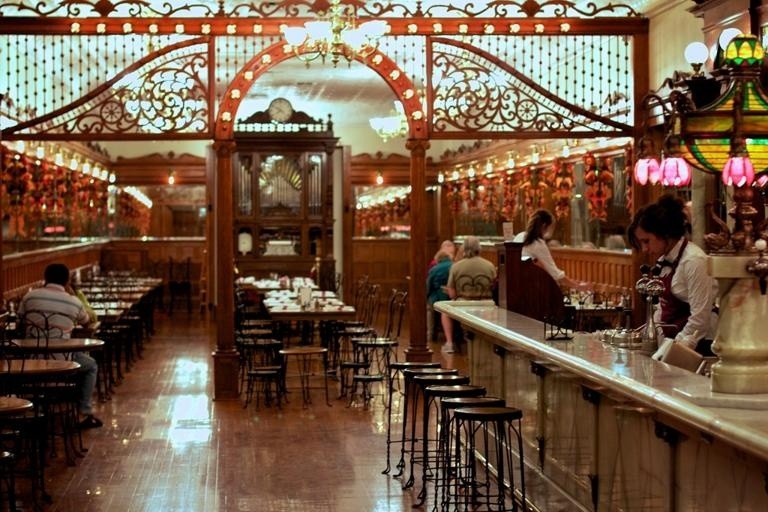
<box><xmin>228</xmin><ymin>109</ymin><xmax>343</xmax><ymax>330</ymax></box>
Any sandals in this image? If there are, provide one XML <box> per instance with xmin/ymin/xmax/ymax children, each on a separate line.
<box><xmin>77</xmin><ymin>414</ymin><xmax>104</xmax><ymax>430</ymax></box>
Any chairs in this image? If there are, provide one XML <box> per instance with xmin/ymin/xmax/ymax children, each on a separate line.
<box><xmin>455</xmin><ymin>273</ymin><xmax>492</xmax><ymax>299</ymax></box>
<box><xmin>563</xmin><ymin>291</ymin><xmax>629</xmax><ymax>332</ymax></box>
<box><xmin>233</xmin><ymin>273</ymin><xmax>409</xmax><ymax>413</ymax></box>
<box><xmin>167</xmin><ymin>255</ymin><xmax>193</xmax><ymax>316</ymax></box>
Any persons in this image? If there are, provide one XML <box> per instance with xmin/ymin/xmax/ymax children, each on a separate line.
<box><xmin>15</xmin><ymin>264</ymin><xmax>103</xmax><ymax>430</ymax></box>
<box><xmin>425</xmin><ymin>236</ymin><xmax>498</xmax><ymax>353</ymax></box>
<box><xmin>513</xmin><ymin>208</ymin><xmax>594</xmax><ymax>292</ymax></box>
<box><xmin>626</xmin><ymin>193</ymin><xmax>719</xmax><ymax>361</ymax></box>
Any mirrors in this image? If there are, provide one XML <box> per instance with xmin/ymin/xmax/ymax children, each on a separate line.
<box><xmin>116</xmin><ymin>180</ymin><xmax>207</xmax><ymax>242</ymax></box>
<box><xmin>1</xmin><ymin>147</ymin><xmax>109</xmax><ymax>257</ymax></box>
<box><xmin>448</xmin><ymin>154</ymin><xmax>633</xmax><ymax>249</ymax></box>
<box><xmin>353</xmin><ymin>181</ymin><xmax>410</xmax><ymax>238</ymax></box>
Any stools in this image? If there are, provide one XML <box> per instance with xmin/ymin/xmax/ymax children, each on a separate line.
<box><xmin>381</xmin><ymin>361</ymin><xmax>528</xmax><ymax>511</ymax></box>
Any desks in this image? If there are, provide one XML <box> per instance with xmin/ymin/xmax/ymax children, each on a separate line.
<box><xmin>0</xmin><ymin>309</ymin><xmax>105</xmax><ymax>510</ymax></box>
<box><xmin>64</xmin><ymin>275</ymin><xmax>163</xmax><ymax>386</ymax></box>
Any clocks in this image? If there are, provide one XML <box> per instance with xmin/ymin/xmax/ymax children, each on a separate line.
<box><xmin>268</xmin><ymin>96</ymin><xmax>293</xmax><ymax>124</ymax></box>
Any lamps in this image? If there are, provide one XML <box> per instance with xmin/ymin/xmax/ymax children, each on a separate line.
<box><xmin>282</xmin><ymin>1</ymin><xmax>392</xmax><ymax>71</ymax></box>
<box><xmin>681</xmin><ymin>37</ymin><xmax>710</xmax><ymax>86</ymax></box>
<box><xmin>368</xmin><ymin>103</ymin><xmax>412</xmax><ymax>145</ymax></box>
<box><xmin>437</xmin><ymin>137</ymin><xmax>610</xmax><ymax>183</ymax></box>
<box><xmin>718</xmin><ymin>27</ymin><xmax>741</xmax><ymax>55</ymax></box>
<box><xmin>14</xmin><ymin>138</ymin><xmax>116</xmax><ymax>182</ymax></box>
<box><xmin>376</xmin><ymin>168</ymin><xmax>387</xmax><ymax>186</ymax></box>
<box><xmin>631</xmin><ymin>33</ymin><xmax>768</xmax><ymax>393</ymax></box>
<box><xmin>167</xmin><ymin>168</ymin><xmax>177</xmax><ymax>185</ymax></box>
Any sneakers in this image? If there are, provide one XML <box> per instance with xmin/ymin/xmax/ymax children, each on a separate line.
<box><xmin>440</xmin><ymin>341</ymin><xmax>457</xmax><ymax>355</ymax></box>
